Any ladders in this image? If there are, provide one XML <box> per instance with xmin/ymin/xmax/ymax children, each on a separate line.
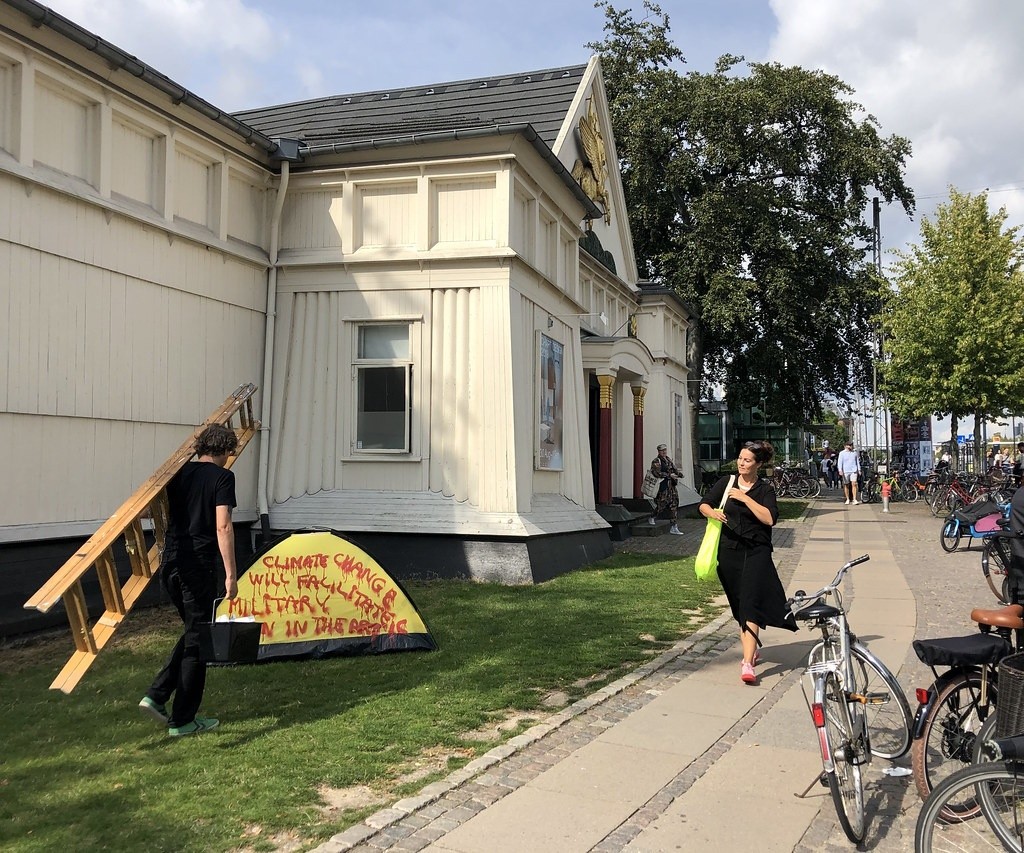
<box><xmin>23</xmin><ymin>381</ymin><xmax>260</xmax><ymax>693</ymax></box>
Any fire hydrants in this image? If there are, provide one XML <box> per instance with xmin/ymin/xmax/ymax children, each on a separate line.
<box><xmin>881</xmin><ymin>481</ymin><xmax>893</xmax><ymax>513</ymax></box>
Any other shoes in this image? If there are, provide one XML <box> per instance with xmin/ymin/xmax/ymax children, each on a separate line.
<box><xmin>852</xmin><ymin>500</ymin><xmax>859</xmax><ymax>505</ymax></box>
<box><xmin>740</xmin><ymin>650</ymin><xmax>759</xmax><ymax>666</ymax></box>
<box><xmin>669</xmin><ymin>524</ymin><xmax>684</xmax><ymax>535</ymax></box>
<box><xmin>845</xmin><ymin>499</ymin><xmax>850</xmax><ymax>505</ymax></box>
<box><xmin>741</xmin><ymin>663</ymin><xmax>756</xmax><ymax>683</ymax></box>
<box><xmin>648</xmin><ymin>516</ymin><xmax>656</xmax><ymax>525</ymax></box>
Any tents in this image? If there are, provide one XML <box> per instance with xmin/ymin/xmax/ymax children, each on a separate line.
<box><xmin>206</xmin><ymin>526</ymin><xmax>440</xmax><ymax>666</ymax></box>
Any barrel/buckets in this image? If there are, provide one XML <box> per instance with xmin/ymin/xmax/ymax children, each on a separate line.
<box><xmin>197</xmin><ymin>598</ymin><xmax>263</xmax><ymax>662</ymax></box>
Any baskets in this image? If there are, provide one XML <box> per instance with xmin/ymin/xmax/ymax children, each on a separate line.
<box><xmin>995</xmin><ymin>653</ymin><xmax>1024</xmax><ymax>739</ymax></box>
<box><xmin>198</xmin><ymin>596</ymin><xmax>264</xmax><ymax>662</ymax></box>
<box><xmin>774</xmin><ymin>467</ymin><xmax>783</xmax><ymax>476</ymax></box>
<box><xmin>940</xmin><ymin>473</ymin><xmax>958</xmax><ymax>485</ymax></box>
<box><xmin>766</xmin><ymin>469</ymin><xmax>773</xmax><ymax>476</ymax></box>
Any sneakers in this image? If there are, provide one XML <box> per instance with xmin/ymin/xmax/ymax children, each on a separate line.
<box><xmin>138</xmin><ymin>697</ymin><xmax>170</xmax><ymax>721</ymax></box>
<box><xmin>168</xmin><ymin>718</ymin><xmax>219</xmax><ymax>736</ymax></box>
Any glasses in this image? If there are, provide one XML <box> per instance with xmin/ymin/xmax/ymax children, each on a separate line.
<box><xmin>229</xmin><ymin>447</ymin><xmax>236</xmax><ymax>456</ymax></box>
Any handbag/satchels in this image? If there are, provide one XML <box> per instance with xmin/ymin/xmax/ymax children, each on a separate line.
<box><xmin>695</xmin><ymin>475</ymin><xmax>735</xmax><ymax>581</ymax></box>
<box><xmin>641</xmin><ymin>458</ymin><xmax>664</xmax><ymax>498</ymax></box>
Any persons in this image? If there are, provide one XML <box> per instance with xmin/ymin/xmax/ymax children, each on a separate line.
<box><xmin>1007</xmin><ymin>486</ymin><xmax>1024</xmax><ymax>655</ymax></box>
<box><xmin>987</xmin><ymin>442</ymin><xmax>1024</xmax><ymax>488</ymax></box>
<box><xmin>696</xmin><ymin>441</ymin><xmax>801</xmax><ymax>684</ymax></box>
<box><xmin>942</xmin><ymin>451</ymin><xmax>952</xmax><ymax>462</ymax></box>
<box><xmin>819</xmin><ymin>442</ymin><xmax>862</xmax><ymax>505</ymax></box>
<box><xmin>137</xmin><ymin>422</ymin><xmax>239</xmax><ymax>737</ymax></box>
<box><xmin>647</xmin><ymin>444</ymin><xmax>684</xmax><ymax>535</ymax></box>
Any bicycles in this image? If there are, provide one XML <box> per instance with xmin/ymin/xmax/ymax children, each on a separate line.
<box><xmin>782</xmin><ymin>553</ymin><xmax>916</xmax><ymax>845</ymax></box>
<box><xmin>859</xmin><ymin>461</ymin><xmax>1024</xmax><ymax>853</ymax></box>
<box><xmin>764</xmin><ymin>459</ymin><xmax>822</xmax><ymax>499</ymax></box>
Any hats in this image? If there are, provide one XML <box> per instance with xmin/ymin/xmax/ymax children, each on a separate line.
<box><xmin>657</xmin><ymin>443</ymin><xmax>667</xmax><ymax>450</ymax></box>
<box><xmin>845</xmin><ymin>441</ymin><xmax>853</xmax><ymax>445</ymax></box>
